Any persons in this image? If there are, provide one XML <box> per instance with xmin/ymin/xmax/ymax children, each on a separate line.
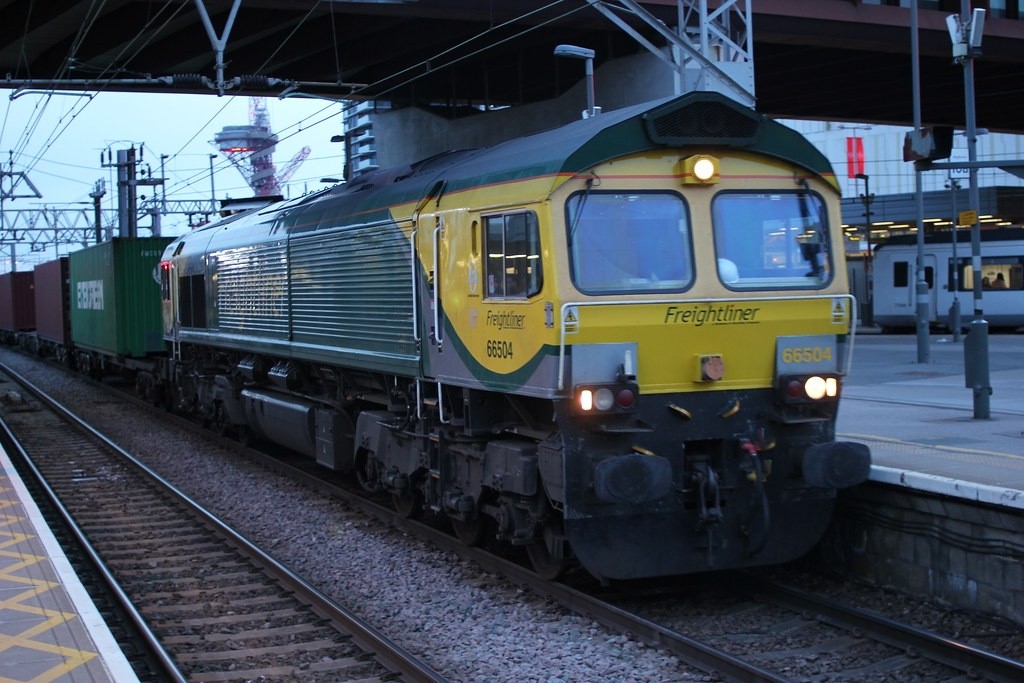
<box><xmin>992</xmin><ymin>273</ymin><xmax>1007</xmax><ymax>289</ymax></box>
<box><xmin>982</xmin><ymin>277</ymin><xmax>991</xmax><ymax>289</ymax></box>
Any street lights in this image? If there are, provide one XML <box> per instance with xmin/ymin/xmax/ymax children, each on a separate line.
<box><xmin>554</xmin><ymin>45</ymin><xmax>596</xmax><ymax>117</ymax></box>
<box><xmin>943</xmin><ymin>177</ymin><xmax>962</xmax><ymax>343</ymax></box>
<box><xmin>854</xmin><ymin>173</ymin><xmax>872</xmax><ymax>262</ymax></box>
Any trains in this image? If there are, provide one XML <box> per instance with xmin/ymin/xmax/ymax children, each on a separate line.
<box><xmin>839</xmin><ymin>225</ymin><xmax>1023</xmax><ymax>335</ymax></box>
<box><xmin>2</xmin><ymin>85</ymin><xmax>873</xmax><ymax>615</ymax></box>
